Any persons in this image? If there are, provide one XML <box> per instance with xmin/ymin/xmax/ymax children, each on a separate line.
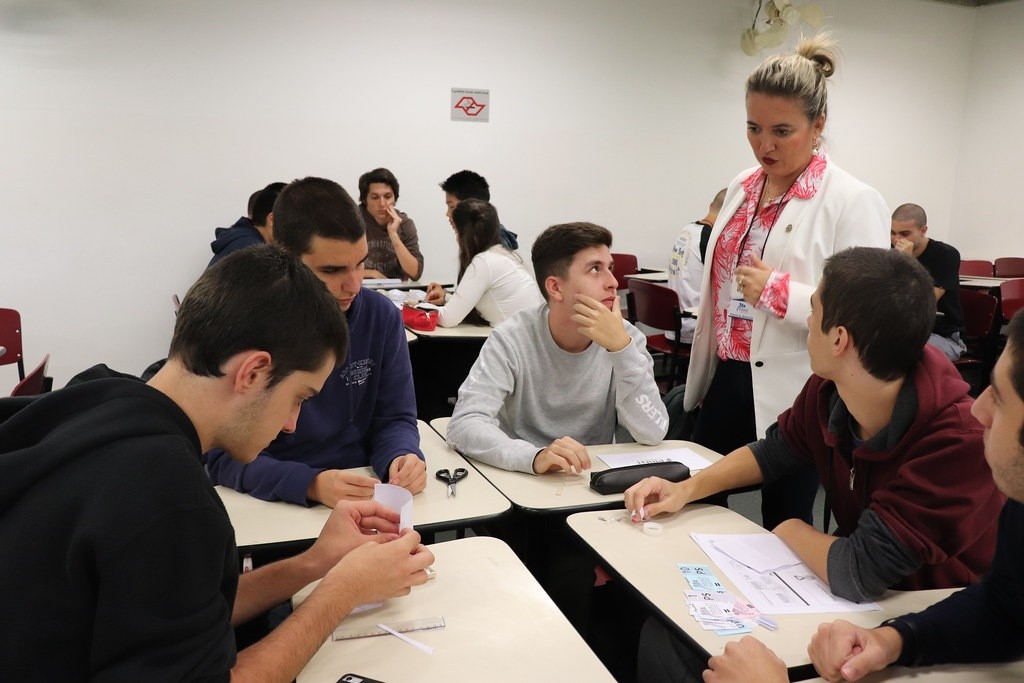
<box><xmin>664</xmin><ymin>188</ymin><xmax>732</xmax><ymax>352</ymax></box>
<box><xmin>357</xmin><ymin>167</ymin><xmax>424</xmax><ymax>282</ymax></box>
<box><xmin>891</xmin><ymin>202</ymin><xmax>967</xmax><ymax>362</ymax></box>
<box><xmin>203</xmin><ymin>182</ymin><xmax>287</xmax><ymax>268</ymax></box>
<box><xmin>684</xmin><ymin>35</ymin><xmax>892</xmax><ymax>456</ymax></box>
<box><xmin>446</xmin><ymin>222</ymin><xmax>670</xmax><ymax>639</ymax></box>
<box><xmin>403</xmin><ymin>169</ymin><xmax>547</xmax><ymax>331</ymax></box>
<box><xmin>623</xmin><ymin>247</ymin><xmax>1010</xmax><ymax>683</ymax></box>
<box><xmin>701</xmin><ymin>303</ymin><xmax>1024</xmax><ymax>683</ymax></box>
<box><xmin>203</xmin><ymin>177</ymin><xmax>427</xmax><ymax>509</ymax></box>
<box><xmin>0</xmin><ymin>245</ymin><xmax>436</xmax><ymax>683</ymax></box>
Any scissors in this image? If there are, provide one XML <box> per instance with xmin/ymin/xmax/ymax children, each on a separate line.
<box><xmin>435</xmin><ymin>467</ymin><xmax>468</xmax><ymax>498</ymax></box>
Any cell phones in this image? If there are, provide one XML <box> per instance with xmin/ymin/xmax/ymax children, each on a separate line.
<box><xmin>336</xmin><ymin>673</ymin><xmax>384</xmax><ymax>683</ymax></box>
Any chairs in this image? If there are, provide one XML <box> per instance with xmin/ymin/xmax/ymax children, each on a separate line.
<box><xmin>610</xmin><ymin>252</ymin><xmax>1024</xmax><ymax>420</ymax></box>
<box><xmin>0</xmin><ymin>307</ymin><xmax>28</xmax><ymax>384</ymax></box>
<box><xmin>11</xmin><ymin>353</ymin><xmax>57</xmax><ymax>401</ymax></box>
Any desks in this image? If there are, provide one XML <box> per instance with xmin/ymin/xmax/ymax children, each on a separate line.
<box><xmin>361</xmin><ymin>278</ymin><xmax>492</xmax><ymax>354</ymax></box>
<box><xmin>959</xmin><ymin>272</ymin><xmax>1024</xmax><ymax>279</ymax></box>
<box><xmin>429</xmin><ymin>409</ymin><xmax>761</xmax><ymax>511</ymax></box>
<box><xmin>567</xmin><ymin>502</ymin><xmax>964</xmax><ymax>669</ymax></box>
<box><xmin>623</xmin><ymin>270</ymin><xmax>669</xmax><ymax>282</ymax></box>
<box><xmin>960</xmin><ymin>278</ymin><xmax>1005</xmax><ymax>287</ymax></box>
<box><xmin>284</xmin><ymin>537</ymin><xmax>614</xmax><ymax>683</ymax></box>
<box><xmin>212</xmin><ymin>417</ymin><xmax>512</xmax><ymax>547</ymax></box>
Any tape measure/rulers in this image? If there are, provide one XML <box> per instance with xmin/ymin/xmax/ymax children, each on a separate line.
<box><xmin>332</xmin><ymin>615</ymin><xmax>445</xmax><ymax>641</ymax></box>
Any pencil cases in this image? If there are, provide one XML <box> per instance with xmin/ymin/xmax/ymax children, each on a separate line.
<box><xmin>589</xmin><ymin>461</ymin><xmax>691</xmax><ymax>495</ymax></box>
<box><xmin>401</xmin><ymin>305</ymin><xmax>439</xmax><ymax>331</ymax></box>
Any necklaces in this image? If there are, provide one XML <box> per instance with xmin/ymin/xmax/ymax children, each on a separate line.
<box><xmin>703</xmin><ymin>218</ymin><xmax>713</xmax><ymax>227</ymax></box>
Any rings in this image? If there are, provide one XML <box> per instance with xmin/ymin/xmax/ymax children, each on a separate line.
<box><xmin>741</xmin><ymin>285</ymin><xmax>743</xmax><ymax>291</ymax></box>
<box><xmin>738</xmin><ymin>275</ymin><xmax>744</xmax><ymax>285</ymax></box>
